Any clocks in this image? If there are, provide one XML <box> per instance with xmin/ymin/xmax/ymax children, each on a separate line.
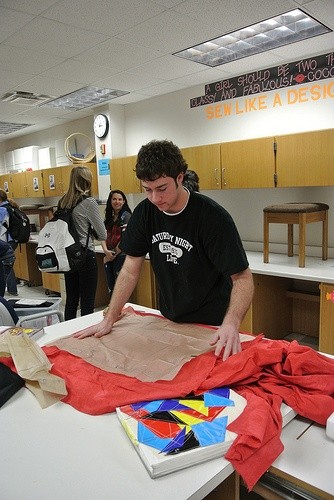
<box><xmin>94</xmin><ymin>114</ymin><xmax>109</xmax><ymax>138</ymax></box>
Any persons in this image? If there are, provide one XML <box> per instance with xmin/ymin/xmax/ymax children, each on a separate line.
<box><xmin>71</xmin><ymin>139</ymin><xmax>255</xmax><ymax>361</ymax></box>
<box><xmin>0</xmin><ymin>189</ymin><xmax>18</xmax><ymax>295</ymax></box>
<box><xmin>101</xmin><ymin>189</ymin><xmax>132</xmax><ymax>302</ymax></box>
<box><xmin>56</xmin><ymin>166</ymin><xmax>108</xmax><ymax>321</ymax></box>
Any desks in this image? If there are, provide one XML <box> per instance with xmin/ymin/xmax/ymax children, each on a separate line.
<box><xmin>0</xmin><ymin>303</ymin><xmax>334</xmax><ymax>500</ymax></box>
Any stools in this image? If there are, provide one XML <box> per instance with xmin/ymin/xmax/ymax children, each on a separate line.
<box><xmin>263</xmin><ymin>202</ymin><xmax>329</xmax><ymax>268</ymax></box>
<box><xmin>19</xmin><ymin>204</ymin><xmax>54</xmax><ymax>231</ymax></box>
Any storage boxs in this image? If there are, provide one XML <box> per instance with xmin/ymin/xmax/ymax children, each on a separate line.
<box><xmin>14</xmin><ymin>297</ymin><xmax>63</xmax><ymax>325</ymax></box>
<box><xmin>0</xmin><ymin>129</ymin><xmax>334</xmax><ymax>198</ymax></box>
<box><xmin>18</xmin><ymin>316</ymin><xmax>48</xmax><ymax>328</ymax></box>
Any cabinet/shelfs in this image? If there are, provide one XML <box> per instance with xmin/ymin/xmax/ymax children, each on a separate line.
<box><xmin>14</xmin><ymin>243</ymin><xmax>334</xmax><ymax>355</ymax></box>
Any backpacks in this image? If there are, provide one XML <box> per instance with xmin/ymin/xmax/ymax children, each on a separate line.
<box><xmin>0</xmin><ymin>203</ymin><xmax>31</xmax><ymax>252</ymax></box>
<box><xmin>34</xmin><ymin>194</ymin><xmax>94</xmax><ymax>275</ymax></box>
